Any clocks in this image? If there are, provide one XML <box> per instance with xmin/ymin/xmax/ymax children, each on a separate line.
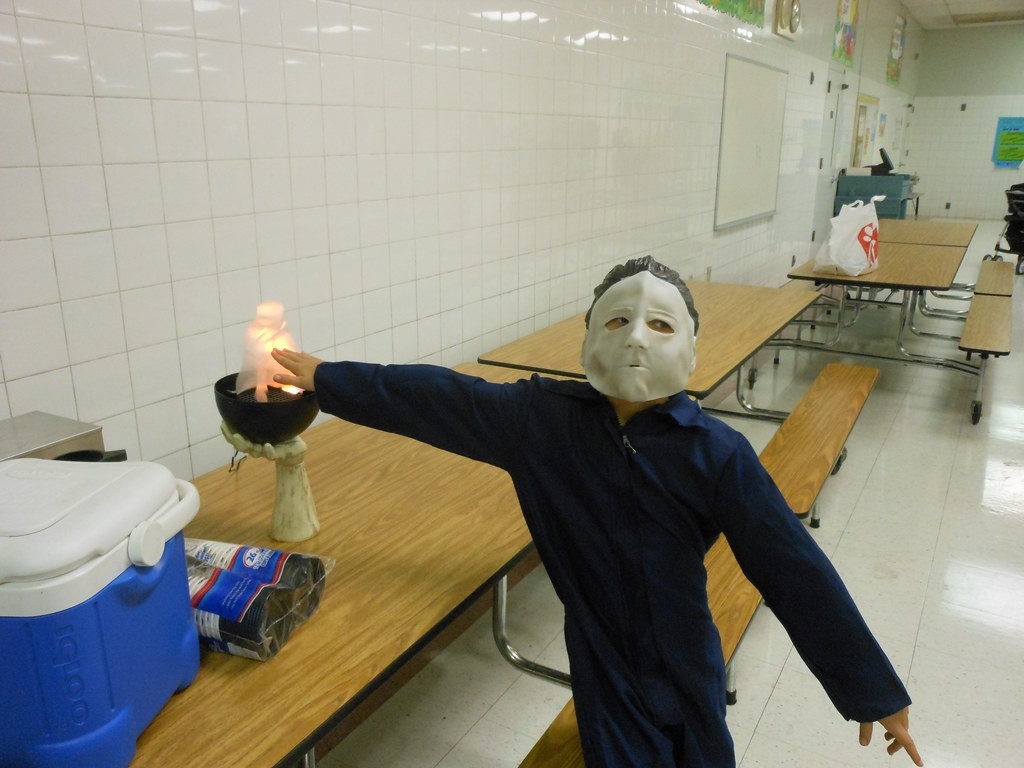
<box><xmin>790</xmin><ymin>0</ymin><xmax>801</xmax><ymax>33</ymax></box>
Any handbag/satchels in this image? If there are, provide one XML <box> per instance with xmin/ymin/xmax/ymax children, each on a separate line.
<box><xmin>812</xmin><ymin>194</ymin><xmax>887</xmax><ymax>277</ymax></box>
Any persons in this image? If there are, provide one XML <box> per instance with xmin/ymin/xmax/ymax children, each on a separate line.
<box><xmin>270</xmin><ymin>255</ymin><xmax>925</xmax><ymax>768</ymax></box>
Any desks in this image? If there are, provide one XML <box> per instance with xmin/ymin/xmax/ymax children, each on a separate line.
<box><xmin>775</xmin><ymin>217</ymin><xmax>987</xmax><ymax>425</ymax></box>
<box><xmin>136</xmin><ymin>362</ymin><xmax>572</xmax><ymax>768</ymax></box>
<box><xmin>905</xmin><ymin>192</ymin><xmax>924</xmax><ymax>214</ymax></box>
<box><xmin>479</xmin><ymin>276</ymin><xmax>824</xmax><ymax>527</ymax></box>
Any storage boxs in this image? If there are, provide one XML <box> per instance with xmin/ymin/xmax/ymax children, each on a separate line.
<box><xmin>0</xmin><ymin>456</ymin><xmax>201</xmax><ymax>768</ymax></box>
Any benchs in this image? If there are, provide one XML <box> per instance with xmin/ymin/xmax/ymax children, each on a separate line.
<box><xmin>974</xmin><ymin>255</ymin><xmax>1014</xmax><ymax>296</ymax></box>
<box><xmin>516</xmin><ymin>363</ymin><xmax>879</xmax><ymax>768</ymax></box>
<box><xmin>958</xmin><ymin>295</ymin><xmax>1016</xmax><ymax>425</ymax></box>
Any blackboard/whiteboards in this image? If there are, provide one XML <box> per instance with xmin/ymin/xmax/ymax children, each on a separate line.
<box><xmin>707</xmin><ymin>52</ymin><xmax>791</xmax><ymax>233</ymax></box>
